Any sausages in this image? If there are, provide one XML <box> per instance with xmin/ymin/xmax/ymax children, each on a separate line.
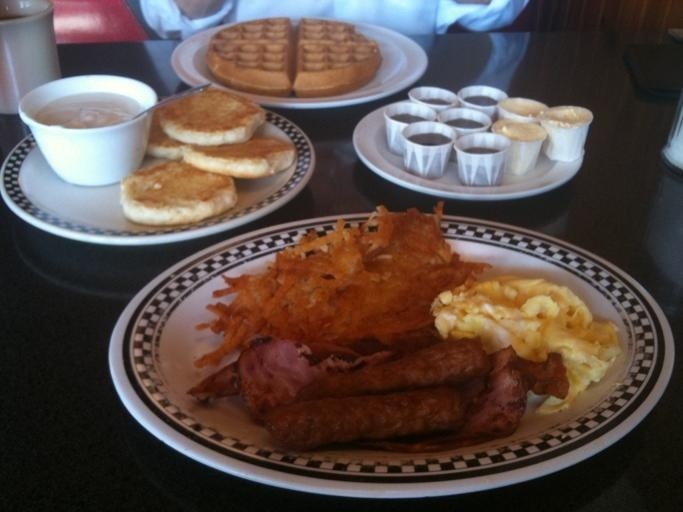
<box><xmin>310</xmin><ymin>339</ymin><xmax>490</xmax><ymax>398</ymax></box>
<box><xmin>263</xmin><ymin>386</ymin><xmax>464</xmax><ymax>454</ymax></box>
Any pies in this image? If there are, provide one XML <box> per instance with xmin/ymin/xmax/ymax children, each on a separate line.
<box><xmin>118</xmin><ymin>162</ymin><xmax>240</xmax><ymax>227</ymax></box>
<box><xmin>147</xmin><ymin>107</ymin><xmax>184</xmax><ymax>162</ymax></box>
<box><xmin>183</xmin><ymin>144</ymin><xmax>294</xmax><ymax>177</ymax></box>
<box><xmin>160</xmin><ymin>85</ymin><xmax>266</xmax><ymax>147</ymax></box>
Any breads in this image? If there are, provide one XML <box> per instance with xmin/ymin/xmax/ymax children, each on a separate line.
<box><xmin>206</xmin><ymin>14</ymin><xmax>382</xmax><ymax>98</ymax></box>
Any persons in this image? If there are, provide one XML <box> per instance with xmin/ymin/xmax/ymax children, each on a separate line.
<box><xmin>141</xmin><ymin>0</ymin><xmax>529</xmax><ymax>39</ymax></box>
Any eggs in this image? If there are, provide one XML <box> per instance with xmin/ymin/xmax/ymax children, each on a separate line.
<box><xmin>433</xmin><ymin>274</ymin><xmax>621</xmax><ymax>417</ymax></box>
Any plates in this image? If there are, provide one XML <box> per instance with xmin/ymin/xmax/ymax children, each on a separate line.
<box><xmin>169</xmin><ymin>16</ymin><xmax>428</xmax><ymax>109</ymax></box>
<box><xmin>352</xmin><ymin>103</ymin><xmax>585</xmax><ymax>201</ymax></box>
<box><xmin>0</xmin><ymin>109</ymin><xmax>315</xmax><ymax>246</ymax></box>
<box><xmin>108</xmin><ymin>212</ymin><xmax>674</xmax><ymax>498</ymax></box>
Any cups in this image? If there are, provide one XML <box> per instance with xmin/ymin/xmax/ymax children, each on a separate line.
<box><xmin>409</xmin><ymin>86</ymin><xmax>459</xmax><ymax>113</ymax></box>
<box><xmin>539</xmin><ymin>106</ymin><xmax>594</xmax><ymax>163</ymax></box>
<box><xmin>490</xmin><ymin>118</ymin><xmax>547</xmax><ymax>176</ymax></box>
<box><xmin>437</xmin><ymin>108</ymin><xmax>492</xmax><ymax>162</ymax></box>
<box><xmin>400</xmin><ymin>121</ymin><xmax>456</xmax><ymax>179</ymax></box>
<box><xmin>456</xmin><ymin>85</ymin><xmax>508</xmax><ymax>119</ymax></box>
<box><xmin>384</xmin><ymin>103</ymin><xmax>437</xmax><ymax>157</ymax></box>
<box><xmin>17</xmin><ymin>74</ymin><xmax>158</xmax><ymax>188</ymax></box>
<box><xmin>453</xmin><ymin>132</ymin><xmax>512</xmax><ymax>186</ymax></box>
<box><xmin>497</xmin><ymin>97</ymin><xmax>549</xmax><ymax>125</ymax></box>
<box><xmin>1</xmin><ymin>0</ymin><xmax>61</xmax><ymax>114</ymax></box>
